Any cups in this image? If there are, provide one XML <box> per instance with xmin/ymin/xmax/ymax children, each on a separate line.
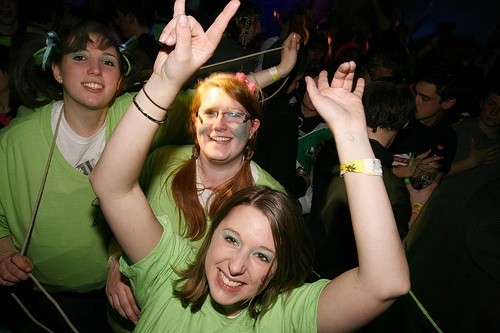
<box><xmin>409</xmin><ymin>161</ymin><xmax>438</xmax><ymax>190</ymax></box>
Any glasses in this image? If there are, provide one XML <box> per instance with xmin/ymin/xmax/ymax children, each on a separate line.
<box><xmin>196</xmin><ymin>110</ymin><xmax>252</xmax><ymax>124</ymax></box>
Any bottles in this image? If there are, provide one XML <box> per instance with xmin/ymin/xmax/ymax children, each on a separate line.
<box><xmin>424</xmin><ymin>145</ymin><xmax>446</xmax><ymax>168</ymax></box>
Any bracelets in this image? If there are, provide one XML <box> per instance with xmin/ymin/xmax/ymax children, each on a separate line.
<box><xmin>269</xmin><ymin>66</ymin><xmax>280</xmax><ymax>81</ymax></box>
<box><xmin>340</xmin><ymin>159</ymin><xmax>383</xmax><ymax>178</ymax></box>
<box><xmin>142</xmin><ymin>84</ymin><xmax>173</xmax><ymax>111</ymax></box>
<box><xmin>133</xmin><ymin>94</ymin><xmax>168</xmax><ymax>125</ymax></box>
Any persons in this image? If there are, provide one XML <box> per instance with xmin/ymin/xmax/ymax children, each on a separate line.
<box><xmin>104</xmin><ymin>71</ymin><xmax>292</xmax><ymax>325</ymax></box>
<box><xmin>0</xmin><ymin>0</ymin><xmax>500</xmax><ymax>333</ymax></box>
<box><xmin>0</xmin><ymin>18</ymin><xmax>301</xmax><ymax>333</ymax></box>
<box><xmin>89</xmin><ymin>0</ymin><xmax>410</xmax><ymax>333</ymax></box>
